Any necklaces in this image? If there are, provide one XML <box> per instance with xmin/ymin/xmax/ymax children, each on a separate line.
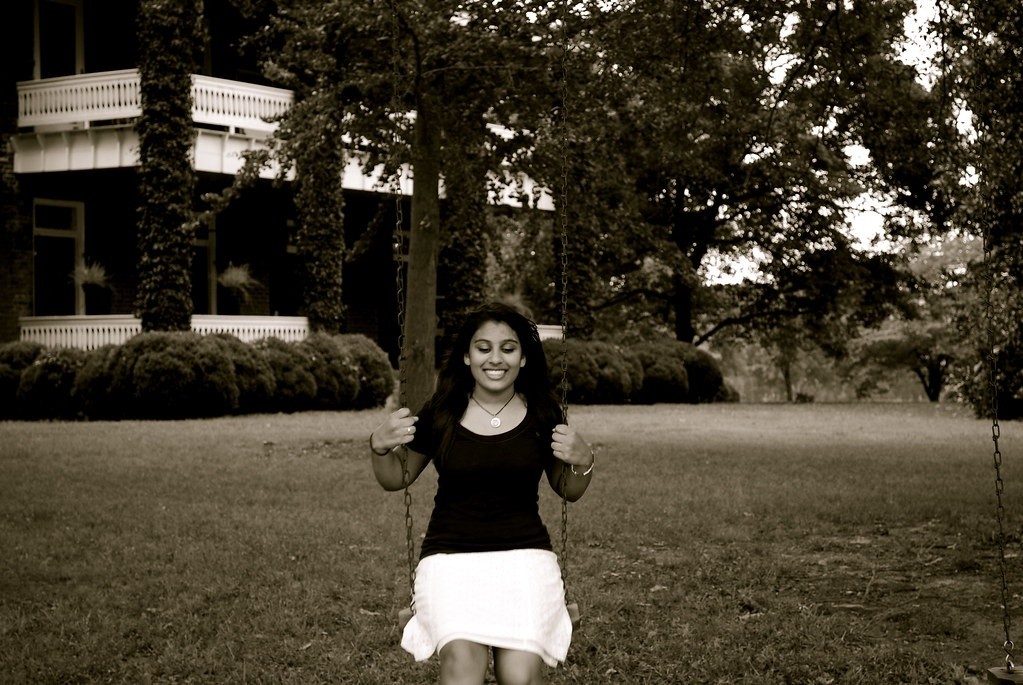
<box><xmin>472</xmin><ymin>392</ymin><xmax>515</xmax><ymax>427</ymax></box>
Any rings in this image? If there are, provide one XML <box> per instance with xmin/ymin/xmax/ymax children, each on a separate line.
<box><xmin>408</xmin><ymin>427</ymin><xmax>411</xmax><ymax>434</ymax></box>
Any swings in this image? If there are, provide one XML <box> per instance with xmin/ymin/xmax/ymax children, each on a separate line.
<box><xmin>391</xmin><ymin>0</ymin><xmax>582</xmax><ymax>636</ymax></box>
<box><xmin>973</xmin><ymin>2</ymin><xmax>1023</xmax><ymax>685</ymax></box>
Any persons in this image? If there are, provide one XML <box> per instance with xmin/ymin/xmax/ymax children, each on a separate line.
<box><xmin>370</xmin><ymin>303</ymin><xmax>595</xmax><ymax>685</ymax></box>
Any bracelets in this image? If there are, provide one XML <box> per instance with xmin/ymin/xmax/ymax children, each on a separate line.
<box><xmin>571</xmin><ymin>450</ymin><xmax>595</xmax><ymax>476</ymax></box>
<box><xmin>370</xmin><ymin>432</ymin><xmax>391</xmax><ymax>456</ymax></box>
